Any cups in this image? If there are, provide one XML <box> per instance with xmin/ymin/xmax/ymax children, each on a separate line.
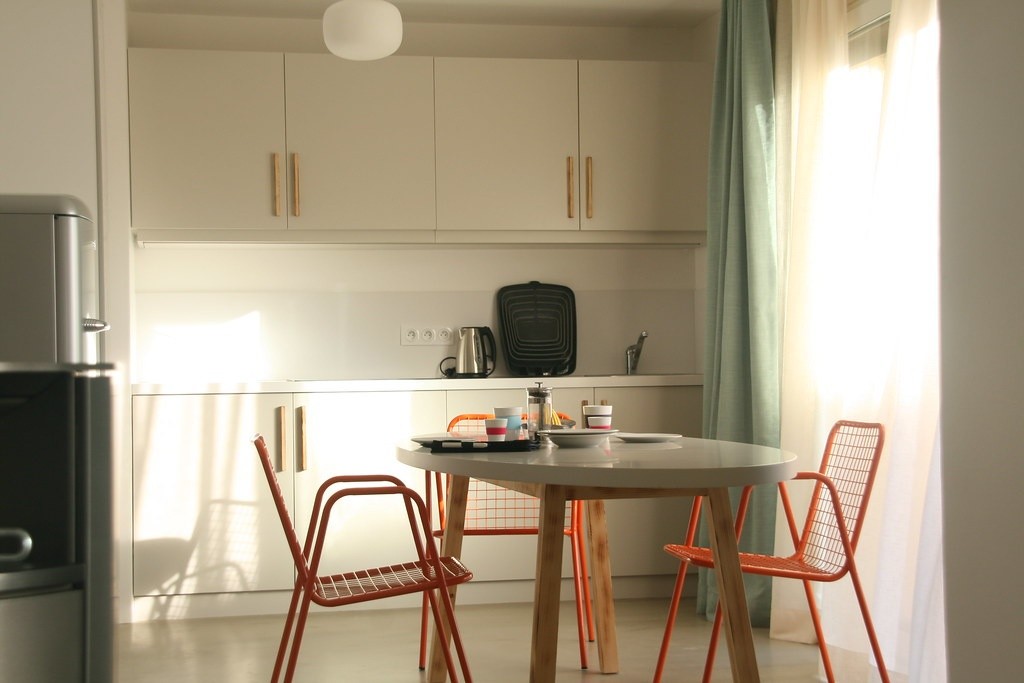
<box><xmin>588</xmin><ymin>417</ymin><xmax>611</xmax><ymax>429</ymax></box>
<box><xmin>494</xmin><ymin>407</ymin><xmax>522</xmax><ymax>441</ymax></box>
<box><xmin>583</xmin><ymin>406</ymin><xmax>612</xmax><ymax>429</ymax></box>
<box><xmin>484</xmin><ymin>418</ymin><xmax>507</xmax><ymax>441</ymax></box>
<box><xmin>527</xmin><ymin>388</ymin><xmax>552</xmax><ymax>443</ymax></box>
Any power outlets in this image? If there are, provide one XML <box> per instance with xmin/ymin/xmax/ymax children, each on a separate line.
<box><xmin>400</xmin><ymin>322</ymin><xmax>454</xmax><ymax>346</ymax></box>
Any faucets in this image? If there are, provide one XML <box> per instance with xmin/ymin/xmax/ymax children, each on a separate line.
<box><xmin>625</xmin><ymin>330</ymin><xmax>649</xmax><ymax>376</ymax></box>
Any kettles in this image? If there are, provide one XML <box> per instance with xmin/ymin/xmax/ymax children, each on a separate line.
<box><xmin>440</xmin><ymin>326</ymin><xmax>496</xmax><ymax>378</ymax></box>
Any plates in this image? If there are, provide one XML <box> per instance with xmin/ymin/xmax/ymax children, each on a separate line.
<box><xmin>616</xmin><ymin>433</ymin><xmax>682</xmax><ymax>443</ymax></box>
<box><xmin>537</xmin><ymin>428</ymin><xmax>618</xmax><ymax>445</ymax></box>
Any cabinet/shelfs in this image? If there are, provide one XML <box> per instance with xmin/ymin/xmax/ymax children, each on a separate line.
<box><xmin>434</xmin><ymin>57</ymin><xmax>708</xmax><ymax>247</ymax></box>
<box><xmin>130</xmin><ymin>390</ymin><xmax>446</xmax><ymax>599</ymax></box>
<box><xmin>126</xmin><ymin>48</ymin><xmax>436</xmax><ymax>242</ymax></box>
<box><xmin>446</xmin><ymin>387</ymin><xmax>701</xmax><ymax>578</ymax></box>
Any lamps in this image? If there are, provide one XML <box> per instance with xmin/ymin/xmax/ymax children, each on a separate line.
<box><xmin>322</xmin><ymin>0</ymin><xmax>401</xmax><ymax>62</ymax></box>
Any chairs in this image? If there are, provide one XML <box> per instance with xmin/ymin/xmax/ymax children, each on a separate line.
<box><xmin>253</xmin><ymin>434</ymin><xmax>475</xmax><ymax>682</ymax></box>
<box><xmin>418</xmin><ymin>410</ymin><xmax>593</xmax><ymax>672</ymax></box>
<box><xmin>652</xmin><ymin>420</ymin><xmax>892</xmax><ymax>683</ymax></box>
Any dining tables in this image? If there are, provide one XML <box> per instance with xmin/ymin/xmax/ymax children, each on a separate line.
<box><xmin>394</xmin><ymin>432</ymin><xmax>799</xmax><ymax>683</ymax></box>
